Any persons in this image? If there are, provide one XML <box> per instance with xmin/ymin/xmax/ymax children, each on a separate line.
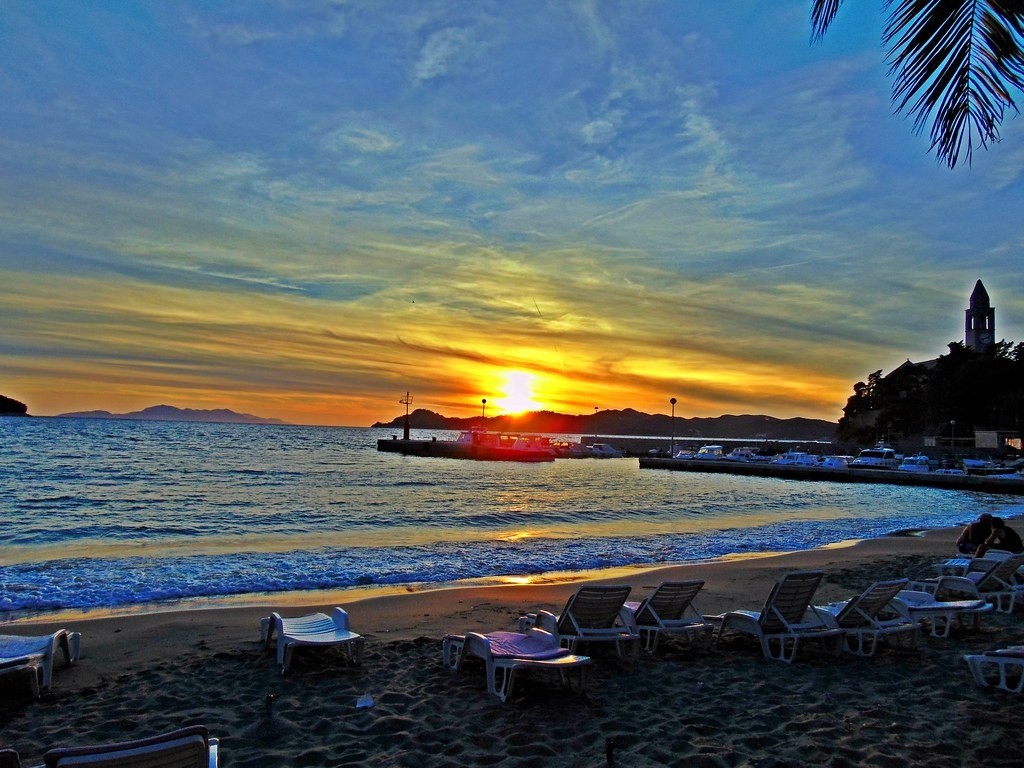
<box><xmin>956</xmin><ymin>513</ymin><xmax>993</xmax><ymax>555</ymax></box>
<box><xmin>974</xmin><ymin>517</ymin><xmax>1023</xmax><ymax>559</ymax></box>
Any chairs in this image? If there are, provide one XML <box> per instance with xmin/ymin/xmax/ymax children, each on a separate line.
<box><xmin>702</xmin><ymin>571</ymin><xmax>847</xmax><ymax>664</ymax></box>
<box><xmin>519</xmin><ymin>585</ymin><xmax>641</xmax><ymax>679</ymax></box>
<box><xmin>931</xmin><ymin>549</ymin><xmax>1024</xmax><ymax>577</ymax></box>
<box><xmin>808</xmin><ymin>578</ymin><xmax>922</xmax><ymax>657</ymax></box>
<box><xmin>44</xmin><ymin>726</ymin><xmax>219</xmax><ymax>768</ymax></box>
<box><xmin>443</xmin><ymin>628</ymin><xmax>591</xmax><ymax>703</ymax></box>
<box><xmin>260</xmin><ymin>607</ymin><xmax>365</xmax><ymax>678</ymax></box>
<box><xmin>623</xmin><ymin>580</ymin><xmax>714</xmax><ymax>655</ymax></box>
<box><xmin>0</xmin><ymin>629</ymin><xmax>82</xmax><ymax>699</ymax></box>
<box><xmin>903</xmin><ymin>553</ymin><xmax>1024</xmax><ymax>613</ymax></box>
<box><xmin>964</xmin><ymin>646</ymin><xmax>1024</xmax><ymax>693</ymax></box>
<box><xmin>880</xmin><ymin>576</ymin><xmax>993</xmax><ymax>637</ymax></box>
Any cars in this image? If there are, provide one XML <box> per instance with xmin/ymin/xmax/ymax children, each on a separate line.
<box><xmin>454</xmin><ymin>431</ymin><xmax>665</xmax><ymax>456</ymax></box>
<box><xmin>674</xmin><ymin>446</ymin><xmax>1020</xmax><ymax>482</ymax></box>
